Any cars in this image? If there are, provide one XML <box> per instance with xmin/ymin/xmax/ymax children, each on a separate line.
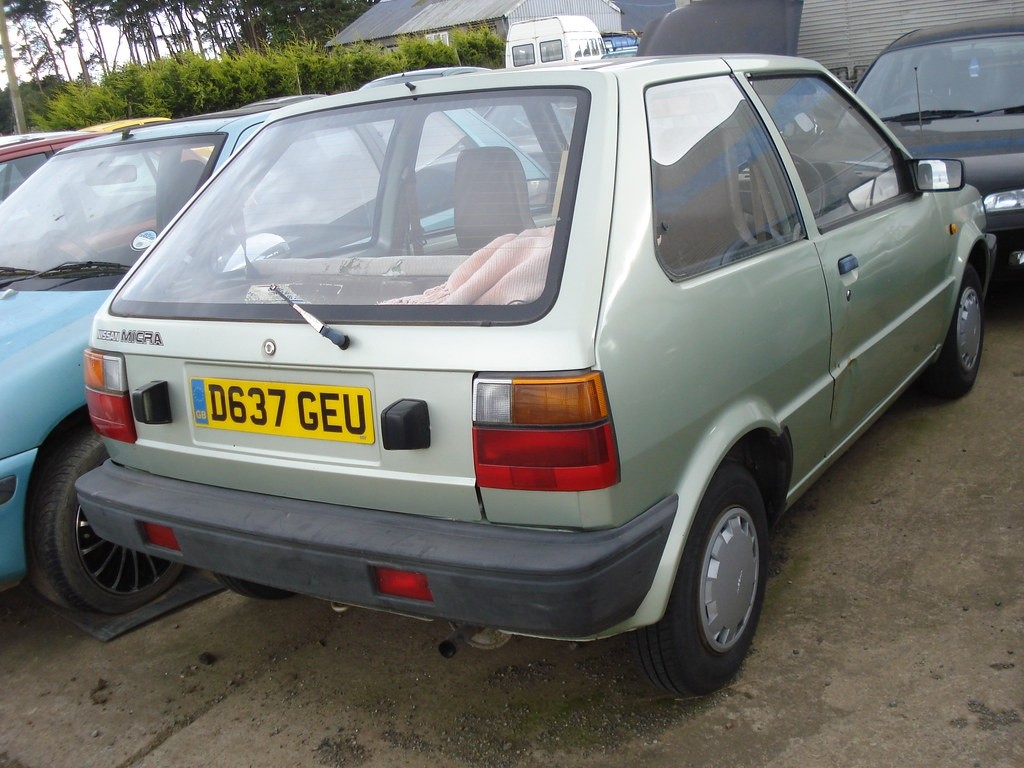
<box><xmin>0</xmin><ymin>67</ymin><xmax>497</xmax><ymax>245</ymax></box>
<box><xmin>0</xmin><ymin>84</ymin><xmax>550</xmax><ymax>615</ymax></box>
<box><xmin>76</xmin><ymin>52</ymin><xmax>999</xmax><ymax>700</ymax></box>
<box><xmin>853</xmin><ymin>16</ymin><xmax>1024</xmax><ymax>287</ymax></box>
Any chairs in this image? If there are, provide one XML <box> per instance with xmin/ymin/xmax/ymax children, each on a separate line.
<box><xmin>654</xmin><ymin>113</ymin><xmax>756</xmax><ymax>265</ymax></box>
<box><xmin>454</xmin><ymin>146</ymin><xmax>537</xmax><ymax>249</ymax></box>
<box><xmin>176</xmin><ymin>160</ymin><xmax>205</xmax><ymax>208</ymax></box>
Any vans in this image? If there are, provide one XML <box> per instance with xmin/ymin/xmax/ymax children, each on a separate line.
<box><xmin>505</xmin><ymin>15</ymin><xmax>639</xmax><ymax>71</ymax></box>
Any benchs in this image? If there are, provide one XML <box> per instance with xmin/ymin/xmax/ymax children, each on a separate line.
<box><xmin>248</xmin><ymin>255</ymin><xmax>469</xmax><ymax>302</ymax></box>
